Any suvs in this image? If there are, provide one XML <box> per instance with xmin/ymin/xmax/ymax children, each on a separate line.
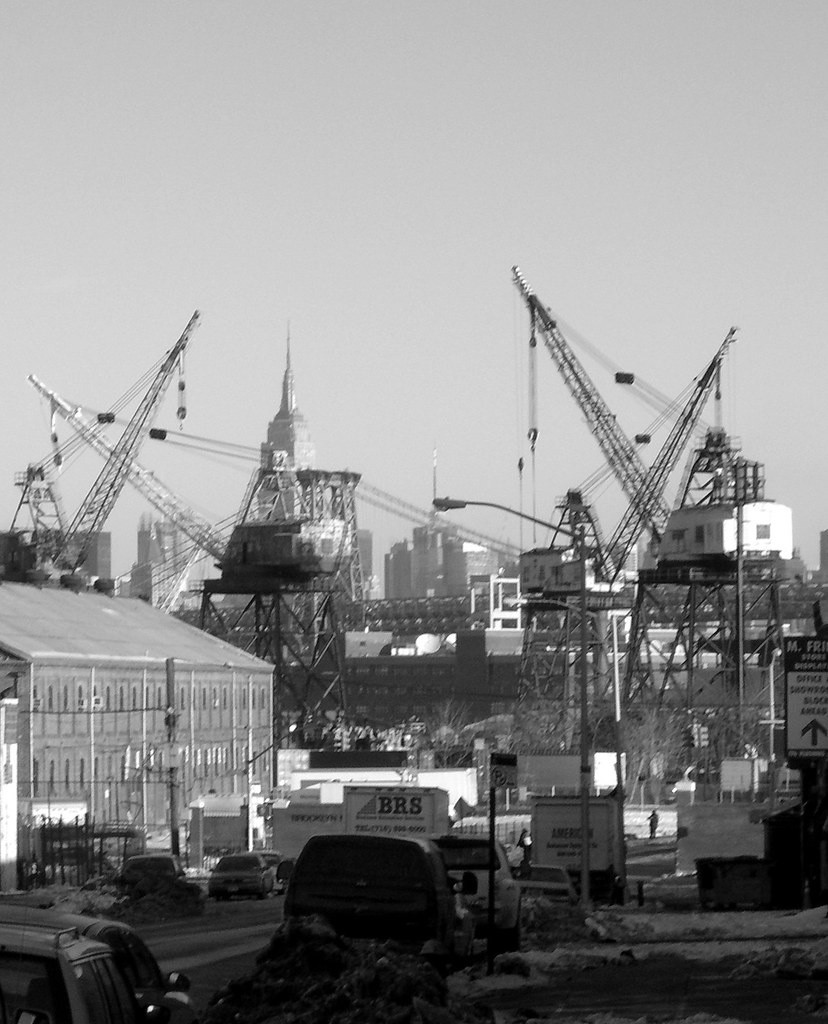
<box><xmin>0</xmin><ymin>908</ymin><xmax>190</xmax><ymax>1024</ymax></box>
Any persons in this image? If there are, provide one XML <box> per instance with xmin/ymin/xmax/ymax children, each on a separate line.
<box><xmin>648</xmin><ymin>810</ymin><xmax>659</xmax><ymax>839</ymax></box>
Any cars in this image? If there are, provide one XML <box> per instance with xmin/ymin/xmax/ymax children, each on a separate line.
<box><xmin>207</xmin><ymin>852</ymin><xmax>275</xmax><ymax>899</ymax></box>
<box><xmin>278</xmin><ymin>832</ymin><xmax>480</xmax><ymax>952</ymax></box>
<box><xmin>436</xmin><ymin>834</ymin><xmax>525</xmax><ymax>952</ymax></box>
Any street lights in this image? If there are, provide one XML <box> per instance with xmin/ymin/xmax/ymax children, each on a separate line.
<box><xmin>433</xmin><ymin>495</ymin><xmax>591</xmax><ymax>905</ymax></box>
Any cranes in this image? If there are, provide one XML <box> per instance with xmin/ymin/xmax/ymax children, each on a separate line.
<box><xmin>17</xmin><ymin>257</ymin><xmax>741</xmax><ymax>758</ymax></box>
